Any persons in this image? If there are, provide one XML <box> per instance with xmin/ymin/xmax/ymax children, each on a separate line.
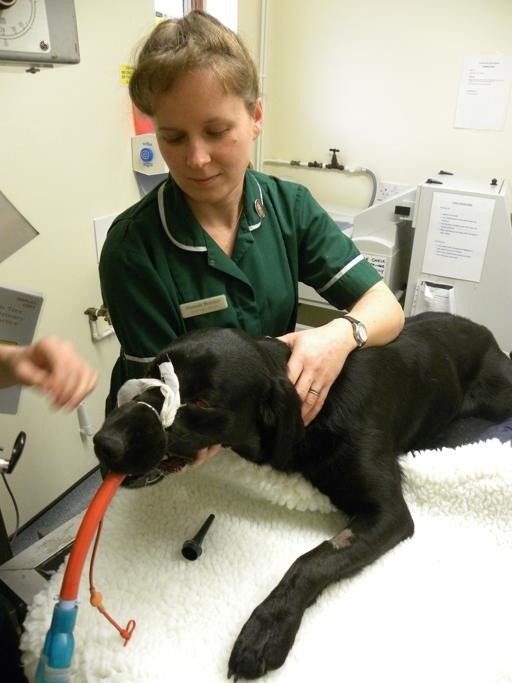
<box><xmin>1</xmin><ymin>335</ymin><xmax>103</xmax><ymax>414</ymax></box>
<box><xmin>97</xmin><ymin>9</ymin><xmax>406</xmax><ymax>485</ymax></box>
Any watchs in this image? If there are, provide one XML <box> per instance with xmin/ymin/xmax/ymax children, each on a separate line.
<box><xmin>342</xmin><ymin>314</ymin><xmax>369</xmax><ymax>352</ymax></box>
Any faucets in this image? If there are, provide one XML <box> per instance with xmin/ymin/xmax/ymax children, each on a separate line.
<box><xmin>326</xmin><ymin>148</ymin><xmax>345</xmax><ymax>170</ymax></box>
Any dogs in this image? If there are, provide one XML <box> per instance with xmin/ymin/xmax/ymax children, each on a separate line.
<box><xmin>91</xmin><ymin>310</ymin><xmax>512</xmax><ymax>683</ymax></box>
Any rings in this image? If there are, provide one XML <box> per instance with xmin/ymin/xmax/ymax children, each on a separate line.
<box><xmin>308</xmin><ymin>386</ymin><xmax>319</xmax><ymax>396</ymax></box>
